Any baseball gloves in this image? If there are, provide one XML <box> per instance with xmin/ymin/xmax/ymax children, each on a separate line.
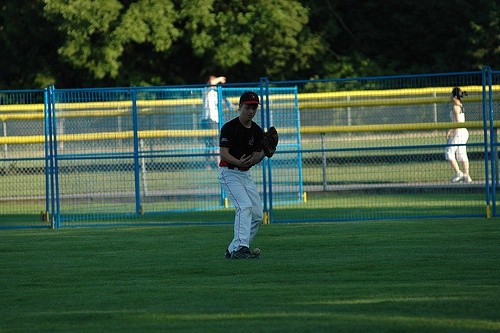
<box><xmin>261</xmin><ymin>127</ymin><xmax>278</xmax><ymax>158</ymax></box>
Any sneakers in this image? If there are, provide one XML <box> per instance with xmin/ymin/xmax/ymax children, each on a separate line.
<box><xmin>451</xmin><ymin>172</ymin><xmax>463</xmax><ymax>181</ymax></box>
<box><xmin>231</xmin><ymin>247</ymin><xmax>256</xmax><ymax>258</ymax></box>
<box><xmin>226</xmin><ymin>249</ymin><xmax>231</xmax><ymax>257</ymax></box>
<box><xmin>463</xmin><ymin>175</ymin><xmax>472</xmax><ymax>182</ymax></box>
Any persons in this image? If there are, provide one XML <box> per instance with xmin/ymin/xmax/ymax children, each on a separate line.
<box><xmin>218</xmin><ymin>92</ymin><xmax>278</xmax><ymax>259</ymax></box>
<box><xmin>444</xmin><ymin>87</ymin><xmax>472</xmax><ymax>184</ymax></box>
<box><xmin>201</xmin><ymin>75</ymin><xmax>236</xmax><ymax>171</ymax></box>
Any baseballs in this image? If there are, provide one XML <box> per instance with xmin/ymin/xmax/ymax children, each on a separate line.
<box><xmin>252</xmin><ymin>248</ymin><xmax>260</xmax><ymax>256</ymax></box>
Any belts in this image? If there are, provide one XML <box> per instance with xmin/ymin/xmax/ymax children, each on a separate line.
<box><xmin>229</xmin><ymin>166</ymin><xmax>241</xmax><ymax>170</ymax></box>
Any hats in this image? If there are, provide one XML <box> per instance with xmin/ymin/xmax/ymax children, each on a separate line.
<box><xmin>240</xmin><ymin>92</ymin><xmax>260</xmax><ymax>104</ymax></box>
